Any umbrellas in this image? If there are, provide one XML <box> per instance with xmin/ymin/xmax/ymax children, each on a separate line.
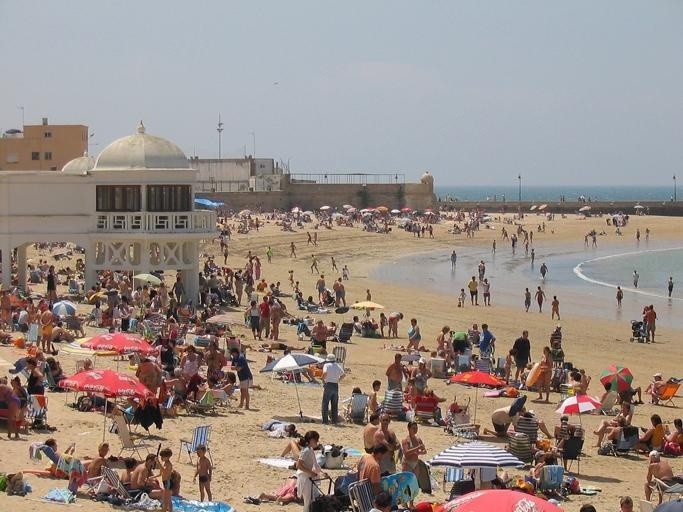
<box><xmin>80</xmin><ymin>332</ymin><xmax>156</xmax><ymax>372</ymax></box>
<box><xmin>425</xmin><ymin>208</ymin><xmax>432</xmax><ymax>212</ymax></box>
<box><xmin>273</xmin><ymin>353</ymin><xmax>325</xmax><ymax>417</ymax></box>
<box><xmin>343</xmin><ymin>204</ymin><xmax>352</xmax><ymax>209</ymax></box>
<box><xmin>552</xmin><ymin>394</ymin><xmax>602</xmax><ymax>426</ymax></box>
<box><xmin>599</xmin><ymin>364</ymin><xmax>634</xmax><ymax>393</ymax></box>
<box><xmin>400</xmin><ymin>355</ymin><xmax>421</xmax><ymax>364</ymax></box>
<box><xmin>529</xmin><ymin>205</ymin><xmax>538</xmax><ymax>210</ymax></box>
<box><xmin>653</xmin><ymin>498</ymin><xmax>683</xmax><ymax>512</ymax></box>
<box><xmin>62</xmin><ymin>337</ymin><xmax>118</xmax><ymax>367</ymax></box>
<box><xmin>53</xmin><ymin>300</ymin><xmax>77</xmax><ymax>320</ymax></box>
<box><xmin>57</xmin><ymin>368</ymin><xmax>153</xmax><ymax>441</ymax></box>
<box><xmin>634</xmin><ymin>205</ymin><xmax>643</xmax><ymax>215</ymax></box>
<box><xmin>391</xmin><ymin>209</ymin><xmax>401</xmax><ymax>214</ymax></box>
<box><xmin>351</xmin><ymin>302</ymin><xmax>384</xmax><ymax>322</ymax></box>
<box><xmin>347</xmin><ymin>208</ymin><xmax>356</xmax><ymax>213</ymax></box>
<box><xmin>539</xmin><ymin>204</ymin><xmax>547</xmax><ymax>210</ymax></box>
<box><xmin>443</xmin><ymin>489</ymin><xmax>562</xmax><ymax>512</ymax></box>
<box><xmin>425</xmin><ymin>439</ymin><xmax>526</xmax><ymax>470</ymax></box>
<box><xmin>194</xmin><ymin>199</ymin><xmax>225</xmax><ymax>207</ymax></box>
<box><xmin>424</xmin><ymin>212</ymin><xmax>434</xmax><ymax>215</ymax></box>
<box><xmin>579</xmin><ymin>206</ymin><xmax>591</xmax><ymax>212</ymax></box>
<box><xmin>450</xmin><ymin>369</ymin><xmax>506</xmax><ymax>424</ymax></box>
<box><xmin>320</xmin><ymin>205</ymin><xmax>330</xmax><ymax>210</ymax></box>
<box><xmin>132</xmin><ymin>273</ymin><xmax>161</xmax><ymax>290</ymax></box>
<box><xmin>401</xmin><ymin>207</ymin><xmax>412</xmax><ymax>218</ymax></box>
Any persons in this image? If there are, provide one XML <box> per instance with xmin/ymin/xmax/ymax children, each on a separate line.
<box><xmin>168</xmin><ymin>291</ymin><xmax>182</xmax><ymax>327</ymax></box>
<box><xmin>319</xmin><ymin>212</ymin><xmax>395</xmax><ymax>235</ymax></box>
<box><xmin>310</xmin><ymin>254</ymin><xmax>319</xmax><ymax>274</ymax></box>
<box><xmin>573</xmin><ymin>373</ymin><xmax>585</xmax><ymax>389</ymax></box>
<box><xmin>284</xmin><ymin>349</ymin><xmax>320</xmax><ymax>384</ymax></box>
<box><xmin>308</xmin><ymin>296</ymin><xmax>328</xmax><ymax>313</ymax></box>
<box><xmin>482</xmin><ymin>262</ymin><xmax>486</xmax><ymax>276</ymax></box>
<box><xmin>249</xmin><ymin>260</ymin><xmax>253</xmax><ymax>268</ymax></box>
<box><xmin>493</xmin><ymin>240</ymin><xmax>496</xmax><ymax>254</ymax></box>
<box><xmin>269</xmin><ymin>297</ymin><xmax>281</xmax><ymax>340</ymax></box>
<box><xmin>640</xmin><ymin>414</ymin><xmax>663</xmax><ymax>442</ymax></box>
<box><xmin>234</xmin><ymin>275</ymin><xmax>243</xmax><ymax>306</ymax></box>
<box><xmin>257</xmin><ymin>279</ymin><xmax>267</xmax><ymax>292</ymax></box>
<box><xmin>231</xmin><ymin>348</ymin><xmax>253</xmax><ymax>410</ymax></box>
<box><xmin>199</xmin><ymin>272</ymin><xmax>209</xmax><ymax>304</ymax></box>
<box><xmin>258</xmin><ymin>481</ymin><xmax>297</xmax><ymax>504</ymax></box>
<box><xmin>645</xmin><ymin>450</ymin><xmax>673</xmax><ymax>501</ymax></box>
<box><xmin>369</xmin><ymin>491</ymin><xmax>393</xmax><ymax>512</ymax></box>
<box><xmin>290</xmin><ymin>318</ymin><xmax>303</xmax><ymax>325</ymax></box>
<box><xmin>388</xmin><ymin>312</ymin><xmax>404</xmax><ymax>338</ymax></box>
<box><xmin>540</xmin><ymin>262</ymin><xmax>548</xmax><ymax>278</ymax></box>
<box><xmin>270</xmin><ymin>284</ymin><xmax>293</xmax><ymax>297</ymax></box>
<box><xmin>69</xmin><ymin>312</ymin><xmax>86</xmax><ymax>338</ymax></box>
<box><xmin>408</xmin><ymin>318</ymin><xmax>421</xmax><ymax>349</ymax></box>
<box><xmin>483</xmin><ymin>406</ymin><xmax>526</xmax><ymax>437</ymax></box>
<box><xmin>451</xmin><ymin>250</ymin><xmax>457</xmax><ymax>272</ymax></box>
<box><xmin>363</xmin><ymin>412</ymin><xmax>380</xmax><ymax>453</ymax></box>
<box><xmin>331</xmin><ymin>257</ymin><xmax>338</xmax><ymax>270</ymax></box>
<box><xmin>259</xmin><ymin>296</ymin><xmax>270</xmax><ymax>338</ymax></box>
<box><xmin>520</xmin><ymin>406</ymin><xmax>554</xmax><ymax>439</ymax></box>
<box><xmin>276</xmin><ymin>282</ymin><xmax>281</xmax><ymax>292</ymax></box>
<box><xmin>136</xmin><ymin>359</ymin><xmax>162</xmax><ymax>394</ymax></box>
<box><xmin>40</xmin><ymin>304</ymin><xmax>54</xmax><ymax>354</ymax></box>
<box><xmin>152</xmin><ymin>448</ymin><xmax>174</xmax><ymax>512</ymax></box>
<box><xmin>293</xmin><ymin>281</ymin><xmax>299</xmax><ymax>293</ymax></box>
<box><xmin>580</xmin><ymin>369</ymin><xmax>591</xmax><ymax>387</ymax></box>
<box><xmin>414</xmin><ymin>387</ymin><xmax>438</xmax><ymax>414</ymax></box>
<box><xmin>374</xmin><ymin>413</ymin><xmax>393</xmax><ymax>470</ymax></box>
<box><xmin>244</xmin><ymin>300</ymin><xmax>260</xmax><ymax>340</ymax></box>
<box><xmin>88</xmin><ymin>443</ymin><xmax>110</xmax><ymax>478</ymax></box>
<box><xmin>386</xmin><ymin>354</ymin><xmax>410</xmax><ymax>392</ymax></box>
<box><xmin>332</xmin><ymin>277</ymin><xmax>346</xmax><ymax>308</ymax></box>
<box><xmin>535</xmin><ymin>286</ymin><xmax>545</xmax><ymax>312</ymax></box>
<box><xmin>325</xmin><ymin>322</ymin><xmax>336</xmax><ymax>333</ymax></box>
<box><xmin>520</xmin><ymin>364</ymin><xmax>533</xmax><ymax>388</ymax></box>
<box><xmin>667</xmin><ymin>419</ymin><xmax>683</xmax><ymax>442</ymax></box>
<box><xmin>297</xmin><ymin>431</ymin><xmax>320</xmax><ymax>512</ymax></box>
<box><xmin>620</xmin><ymin>496</ymin><xmax>633</xmax><ymax>512</ymax></box>
<box><xmin>202</xmin><ymin>329</ymin><xmax>215</xmax><ymax>346</ymax></box>
<box><xmin>366</xmin><ymin>289</ymin><xmax>372</xmax><ymax>301</ymax></box>
<box><xmin>298</xmin><ymin>292</ymin><xmax>304</xmax><ymax>303</ymax></box>
<box><xmin>26</xmin><ymin>303</ymin><xmax>38</xmax><ymax>324</ymax></box>
<box><xmin>353</xmin><ymin>316</ymin><xmax>363</xmax><ymax>332</ymax></box>
<box><xmin>316</xmin><ymin>275</ymin><xmax>325</xmax><ymax>300</ymax></box>
<box><xmin>312</xmin><ymin>319</ymin><xmax>328</xmax><ymax>349</ymax></box>
<box><xmin>345</xmin><ymin>265</ymin><xmax>349</xmax><ymax>271</ymax></box>
<box><xmin>282</xmin><ymin>440</ymin><xmax>305</xmax><ymax>460</ymax></box>
<box><xmin>439</xmin><ymin>207</ymin><xmax>501</xmax><ymax>238</ymax></box>
<box><xmin>52</xmin><ymin>321</ymin><xmax>74</xmax><ymax>342</ymax></box>
<box><xmin>172</xmin><ymin>278</ymin><xmax>186</xmax><ymax>302</ymax></box>
<box><xmin>359</xmin><ymin>443</ymin><xmax>388</xmax><ymax>495</ymax></box>
<box><xmin>307</xmin><ymin>232</ymin><xmax>314</xmax><ymax>245</ymax></box>
<box><xmin>643</xmin><ymin>306</ymin><xmax>649</xmax><ymax>329</ymax></box>
<box><xmin>181</xmin><ymin>346</ymin><xmax>201</xmax><ymax>401</ymax></box>
<box><xmin>616</xmin><ymin>286</ymin><xmax>623</xmax><ymax>306</ymax></box>
<box><xmin>646</xmin><ymin>228</ymin><xmax>650</xmax><ymax>239</ymax></box>
<box><xmin>224</xmin><ymin>245</ymin><xmax>229</xmax><ymax>265</ymax></box>
<box><xmin>36</xmin><ymin>300</ymin><xmax>45</xmax><ymax>350</ymax></box>
<box><xmin>645</xmin><ymin>373</ymin><xmax>663</xmax><ymax>404</ymax></box>
<box><xmin>48</xmin><ymin>357</ymin><xmax>64</xmax><ymax>384</ymax></box>
<box><xmin>619</xmin><ymin>386</ymin><xmax>645</xmax><ymax>404</ymax></box>
<box><xmin>119</xmin><ymin>398</ymin><xmax>140</xmax><ymax>424</ymax></box>
<box><xmin>291</xmin><ymin>270</ymin><xmax>294</xmax><ymax>284</ymax></box>
<box><xmin>343</xmin><ymin>268</ymin><xmax>349</xmax><ymax>280</ymax></box>
<box><xmin>608</xmin><ymin>416</ymin><xmax>632</xmax><ymax>452</ymax></box>
<box><xmin>121</xmin><ymin>458</ymin><xmax>137</xmax><ymax>487</ymax></box>
<box><xmin>0</xmin><ymin>240</ymin><xmax>87</xmax><ymax>300</ymax></box>
<box><xmin>537</xmin><ymin>346</ymin><xmax>552</xmax><ymax>404</ymax></box>
<box><xmin>480</xmin><ymin>324</ymin><xmax>496</xmax><ymax>358</ymax></box>
<box><xmin>585</xmin><ymin>228</ymin><xmax>623</xmax><ymax>249</ymax></box>
<box><xmin>245</xmin><ymin>263</ymin><xmax>251</xmax><ymax>276</ymax></box>
<box><xmin>400</xmin><ymin>219</ymin><xmax>436</xmax><ymax>240</ymax></box>
<box><xmin>174</xmin><ymin>367</ymin><xmax>186</xmax><ymax>384</ymax></box>
<box><xmin>513</xmin><ymin>330</ymin><xmax>531</xmax><ymax>383</ymax></box>
<box><xmin>504</xmin><ymin>348</ymin><xmax>517</xmax><ymax>385</ymax></box>
<box><xmin>436</xmin><ymin>326</ymin><xmax>450</xmax><ymax>358</ymax></box>
<box><xmin>379</xmin><ymin>312</ymin><xmax>388</xmax><ymax>338</ymax></box>
<box><xmin>437</xmin><ymin>350</ymin><xmax>451</xmax><ymax>368</ymax></box>
<box><xmin>343</xmin><ymin>387</ymin><xmax>363</xmax><ymax>402</ymax></box>
<box><xmin>524</xmin><ymin>288</ymin><xmax>532</xmax><ymax>312</ymax></box>
<box><xmin>245</xmin><ymin>251</ymin><xmax>257</xmax><ymax>264</ymax></box>
<box><xmin>632</xmin><ymin>270</ymin><xmax>640</xmax><ymax>287</ymax></box>
<box><xmin>452</xmin><ymin>331</ymin><xmax>469</xmax><ymax>355</ymax></box>
<box><xmin>534</xmin><ymin>452</ymin><xmax>556</xmax><ymax>478</ymax></box>
<box><xmin>644</xmin><ymin>304</ymin><xmax>657</xmax><ymax>343</ymax></box>
<box><xmin>637</xmin><ymin>229</ymin><xmax>640</xmax><ymax>241</ymax></box>
<box><xmin>0</xmin><ymin>290</ymin><xmax>15</xmax><ymax>332</ymax></box>
<box><xmin>557</xmin><ymin>425</ymin><xmax>581</xmax><ymax>470</ymax></box>
<box><xmin>531</xmin><ymin>249</ymin><xmax>535</xmax><ymax>264</ymax></box>
<box><xmin>478</xmin><ymin>261</ymin><xmax>483</xmax><ymax>282</ymax></box>
<box><xmin>220</xmin><ymin>237</ymin><xmax>224</xmax><ymax>252</ymax></box>
<box><xmin>289</xmin><ymin>242</ymin><xmax>296</xmax><ymax>258</ymax></box>
<box><xmin>322</xmin><ymin>354</ymin><xmax>346</xmax><ymax>425</ymax></box>
<box><xmin>235</xmin><ymin>270</ymin><xmax>242</xmax><ymax>280</ymax></box>
<box><xmin>222</xmin><ymin>266</ymin><xmax>234</xmax><ymax>284</ymax></box>
<box><xmin>668</xmin><ymin>277</ymin><xmax>674</xmax><ymax>297</ymax></box>
<box><xmin>210</xmin><ymin>274</ymin><xmax>223</xmax><ymax>305</ymax></box>
<box><xmin>255</xmin><ymin>259</ymin><xmax>261</xmax><ymax>280</ymax></box>
<box><xmin>287</xmin><ymin>271</ymin><xmax>292</xmax><ymax>284</ymax></box>
<box><xmin>410</xmin><ymin>357</ymin><xmax>447</xmax><ymax>403</ymax></box>
<box><xmin>18</xmin><ymin>307</ymin><xmax>30</xmax><ymax>331</ymax></box>
<box><xmin>203</xmin><ymin>341</ymin><xmax>227</xmax><ymax>389</ymax></box>
<box><xmin>470</xmin><ymin>324</ymin><xmax>481</xmax><ymax>344</ymax></box>
<box><xmin>11</xmin><ymin>376</ymin><xmax>27</xmax><ymax>408</ymax></box>
<box><xmin>427</xmin><ymin>351</ymin><xmax>437</xmax><ymax>369</ymax></box>
<box><xmin>671</xmin><ymin>195</ymin><xmax>673</xmax><ymax>201</ymax></box>
<box><xmin>45</xmin><ymin>438</ymin><xmax>76</xmax><ymax>458</ymax></box>
<box><xmin>256</xmin><ymin>420</ymin><xmax>296</xmax><ymax>438</ymax></box>
<box><xmin>368</xmin><ymin>380</ymin><xmax>381</xmax><ymax>416</ymax></box>
<box><xmin>552</xmin><ymin>295</ymin><xmax>560</xmax><ymax>320</ymax></box>
<box><xmin>592</xmin><ymin>401</ymin><xmax>631</xmax><ymax>447</ymax></box>
<box><xmin>458</xmin><ymin>288</ymin><xmax>467</xmax><ymax>307</ymax></box>
<box><xmin>468</xmin><ymin>276</ymin><xmax>479</xmax><ymax>305</ymax></box>
<box><xmin>266</xmin><ymin>246</ymin><xmax>273</xmax><ymax>263</ymax></box>
<box><xmin>220</xmin><ymin>372</ymin><xmax>236</xmax><ymax>395</ymax></box>
<box><xmin>401</xmin><ymin>422</ymin><xmax>428</xmax><ymax>511</ymax></box>
<box><xmin>193</xmin><ymin>444</ymin><xmax>212</xmax><ymax>502</ymax></box>
<box><xmin>550</xmin><ymin>325</ymin><xmax>563</xmax><ymax>368</ymax></box>
<box><xmin>599</xmin><ymin>382</ymin><xmax>612</xmax><ymax>403</ymax></box>
<box><xmin>313</xmin><ymin>232</ymin><xmax>317</xmax><ymax>245</ymax></box>
<box><xmin>580</xmin><ymin>504</ymin><xmax>596</xmax><ymax>512</ymax></box>
<box><xmin>596</xmin><ymin>211</ymin><xmax>630</xmax><ymax>227</ymax></box>
<box><xmin>26</xmin><ymin>359</ymin><xmax>45</xmax><ymax>395</ymax></box>
<box><xmin>244</xmin><ymin>276</ymin><xmax>255</xmax><ymax>302</ymax></box>
<box><xmin>267</xmin><ymin>207</ymin><xmax>319</xmax><ymax>231</ymax></box>
<box><xmin>283</xmin><ymin>316</ymin><xmax>309</xmax><ymax>323</ymax></box>
<box><xmin>130</xmin><ymin>454</ymin><xmax>163</xmax><ymax>499</ymax></box>
<box><xmin>216</xmin><ymin>200</ymin><xmax>266</xmax><ymax>236</ymax></box>
<box><xmin>554</xmin><ymin>414</ymin><xmax>569</xmax><ymax>440</ymax></box>
<box><xmin>0</xmin><ymin>376</ymin><xmax>12</xmax><ymax>408</ymax></box>
<box><xmin>87</xmin><ymin>266</ymin><xmax>132</xmax><ymax>332</ymax></box>
<box><xmin>482</xmin><ymin>279</ymin><xmax>491</xmax><ymax>306</ymax></box>
<box><xmin>262</xmin><ymin>342</ymin><xmax>305</xmax><ymax>351</ymax></box>
<box><xmin>128</xmin><ymin>355</ymin><xmax>139</xmax><ymax>371</ymax></box>
<box><xmin>501</xmin><ymin>212</ymin><xmax>567</xmax><ymax>250</ymax></box>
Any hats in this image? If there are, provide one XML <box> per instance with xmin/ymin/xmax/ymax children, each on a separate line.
<box><xmin>326</xmin><ymin>353</ymin><xmax>337</xmax><ymax>362</ymax></box>
<box><xmin>418</xmin><ymin>356</ymin><xmax>427</xmax><ymax>365</ymax></box>
<box><xmin>653</xmin><ymin>372</ymin><xmax>662</xmax><ymax>377</ymax></box>
